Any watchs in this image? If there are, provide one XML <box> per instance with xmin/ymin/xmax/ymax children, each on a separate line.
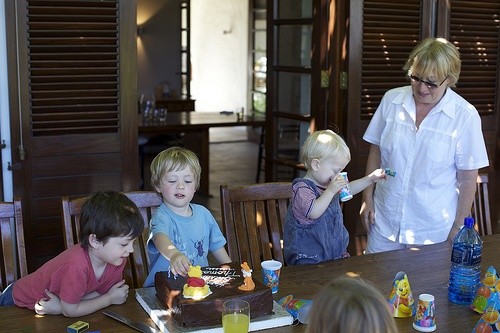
<box><xmin>453</xmin><ymin>222</ymin><xmax>465</xmax><ymax>230</ymax></box>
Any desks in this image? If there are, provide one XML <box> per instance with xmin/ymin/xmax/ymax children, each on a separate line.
<box><xmin>0</xmin><ymin>234</ymin><xmax>500</xmax><ymax>333</ymax></box>
<box><xmin>138</xmin><ymin>110</ymin><xmax>267</xmax><ymax>197</ymax></box>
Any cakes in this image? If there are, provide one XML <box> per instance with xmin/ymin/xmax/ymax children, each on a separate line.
<box><xmin>154</xmin><ymin>261</ymin><xmax>275</xmax><ymax>329</ymax></box>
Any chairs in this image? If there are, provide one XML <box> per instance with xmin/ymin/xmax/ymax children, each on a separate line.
<box><xmin>219</xmin><ymin>182</ymin><xmax>293</xmax><ymax>271</ymax></box>
<box><xmin>0</xmin><ymin>200</ymin><xmax>29</xmax><ymax>293</ymax></box>
<box><xmin>468</xmin><ymin>174</ymin><xmax>492</xmax><ymax>237</ymax></box>
<box><xmin>255</xmin><ymin>124</ymin><xmax>300</xmax><ymax>184</ymax></box>
<box><xmin>137</xmin><ymin>99</ymin><xmax>196</xmax><ymax>191</ymax></box>
<box><xmin>61</xmin><ymin>192</ymin><xmax>163</xmax><ymax>290</ymax></box>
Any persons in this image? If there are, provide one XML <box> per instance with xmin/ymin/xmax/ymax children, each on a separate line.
<box><xmin>305</xmin><ymin>275</ymin><xmax>400</xmax><ymax>333</ymax></box>
<box><xmin>359</xmin><ymin>37</ymin><xmax>489</xmax><ymax>256</ymax></box>
<box><xmin>0</xmin><ymin>190</ymin><xmax>145</xmax><ymax>318</ymax></box>
<box><xmin>283</xmin><ymin>125</ymin><xmax>388</xmax><ymax>267</ymax></box>
<box><xmin>142</xmin><ymin>147</ymin><xmax>232</xmax><ymax>287</ymax></box>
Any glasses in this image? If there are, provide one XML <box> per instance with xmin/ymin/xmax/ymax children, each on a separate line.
<box><xmin>410</xmin><ymin>73</ymin><xmax>449</xmax><ymax>88</ymax></box>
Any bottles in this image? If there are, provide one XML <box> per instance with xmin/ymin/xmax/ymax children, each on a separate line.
<box><xmin>448</xmin><ymin>216</ymin><xmax>483</xmax><ymax>305</ymax></box>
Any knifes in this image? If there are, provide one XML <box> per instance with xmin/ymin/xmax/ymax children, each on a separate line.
<box><xmin>102</xmin><ymin>309</ymin><xmax>159</xmax><ymax>333</ymax></box>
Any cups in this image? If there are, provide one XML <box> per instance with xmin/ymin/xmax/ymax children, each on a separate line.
<box><xmin>140</xmin><ymin>94</ymin><xmax>156</xmax><ymax>122</ymax></box>
<box><xmin>221</xmin><ymin>300</ymin><xmax>250</xmax><ymax>333</ymax></box>
<box><xmin>261</xmin><ymin>260</ymin><xmax>282</xmax><ymax>294</ymax></box>
<box><xmin>236</xmin><ymin>108</ymin><xmax>245</xmax><ymax>120</ymax></box>
<box><xmin>331</xmin><ymin>171</ymin><xmax>354</xmax><ymax>202</ymax></box>
<box><xmin>412</xmin><ymin>293</ymin><xmax>437</xmax><ymax>332</ymax></box>
